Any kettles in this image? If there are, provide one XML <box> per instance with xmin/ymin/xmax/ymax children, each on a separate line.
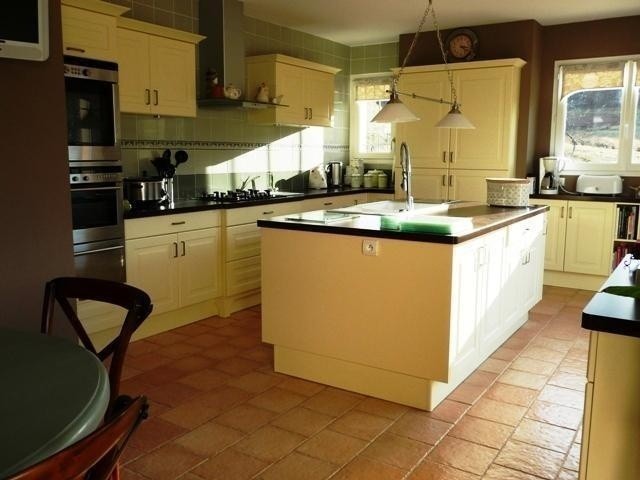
<box><xmin>327</xmin><ymin>162</ymin><xmax>341</xmax><ymax>189</ymax></box>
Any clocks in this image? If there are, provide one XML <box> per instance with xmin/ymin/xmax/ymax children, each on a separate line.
<box><xmin>445</xmin><ymin>29</ymin><xmax>479</xmax><ymax>63</ymax></box>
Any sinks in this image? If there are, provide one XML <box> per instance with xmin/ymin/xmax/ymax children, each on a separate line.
<box><xmin>327</xmin><ymin>199</ymin><xmax>448</xmax><ymax>217</ymax></box>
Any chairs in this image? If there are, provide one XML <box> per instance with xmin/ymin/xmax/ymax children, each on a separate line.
<box><xmin>0</xmin><ymin>393</ymin><xmax>150</xmax><ymax>479</ymax></box>
<box><xmin>40</xmin><ymin>277</ymin><xmax>155</xmax><ymax>415</ymax></box>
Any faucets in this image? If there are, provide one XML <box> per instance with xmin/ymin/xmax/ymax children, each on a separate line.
<box><xmin>399</xmin><ymin>142</ymin><xmax>412</xmax><ymax>208</ymax></box>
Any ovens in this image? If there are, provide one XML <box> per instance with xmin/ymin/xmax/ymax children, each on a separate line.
<box><xmin>62</xmin><ymin>54</ymin><xmax>122</xmax><ymax>161</ymax></box>
<box><xmin>69</xmin><ymin>161</ymin><xmax>127</xmax><ymax>301</ymax></box>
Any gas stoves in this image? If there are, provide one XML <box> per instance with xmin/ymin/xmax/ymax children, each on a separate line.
<box><xmin>199</xmin><ymin>189</ymin><xmax>286</xmax><ymax>202</ymax></box>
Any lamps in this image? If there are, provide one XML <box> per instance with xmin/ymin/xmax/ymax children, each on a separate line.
<box><xmin>370</xmin><ymin>0</ymin><xmax>475</xmax><ymax>130</ymax></box>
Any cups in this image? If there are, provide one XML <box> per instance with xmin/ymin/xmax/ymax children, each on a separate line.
<box><xmin>351</xmin><ymin>169</ymin><xmax>387</xmax><ymax>188</ymax></box>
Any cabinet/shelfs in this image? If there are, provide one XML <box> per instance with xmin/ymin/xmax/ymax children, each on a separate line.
<box><xmin>128</xmin><ymin>208</ymin><xmax>223</xmax><ymax>341</ymax></box>
<box><xmin>224</xmin><ymin>167</ymin><xmax>552</xmax><ymax>411</ymax></box>
<box><xmin>60</xmin><ymin>0</ymin><xmax>132</xmax><ymax>63</ymax></box>
<box><xmin>580</xmin><ymin>256</ymin><xmax>639</xmax><ymax>480</ymax></box>
<box><xmin>388</xmin><ymin>57</ymin><xmax>526</xmax><ymax>167</ymax></box>
<box><xmin>245</xmin><ymin>55</ymin><xmax>342</xmax><ymax>128</ymax></box>
<box><xmin>116</xmin><ymin>15</ymin><xmax>208</xmax><ymax>119</ymax></box>
<box><xmin>530</xmin><ymin>196</ymin><xmax>639</xmax><ymax>291</ymax></box>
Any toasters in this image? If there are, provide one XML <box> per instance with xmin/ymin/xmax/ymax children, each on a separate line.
<box><xmin>577</xmin><ymin>173</ymin><xmax>623</xmax><ymax>197</ymax></box>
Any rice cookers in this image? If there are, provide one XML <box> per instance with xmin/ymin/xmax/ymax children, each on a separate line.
<box><xmin>122</xmin><ymin>171</ymin><xmax>168</xmax><ymax>212</ymax></box>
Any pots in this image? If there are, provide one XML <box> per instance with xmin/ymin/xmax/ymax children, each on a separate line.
<box><xmin>484</xmin><ymin>177</ymin><xmax>530</xmax><ymax>207</ymax></box>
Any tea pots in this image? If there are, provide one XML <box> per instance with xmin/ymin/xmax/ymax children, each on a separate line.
<box><xmin>224</xmin><ymin>83</ymin><xmax>242</xmax><ymax>100</ymax></box>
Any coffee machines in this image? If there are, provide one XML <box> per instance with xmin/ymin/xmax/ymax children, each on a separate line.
<box><xmin>540</xmin><ymin>157</ymin><xmax>558</xmax><ymax>195</ymax></box>
